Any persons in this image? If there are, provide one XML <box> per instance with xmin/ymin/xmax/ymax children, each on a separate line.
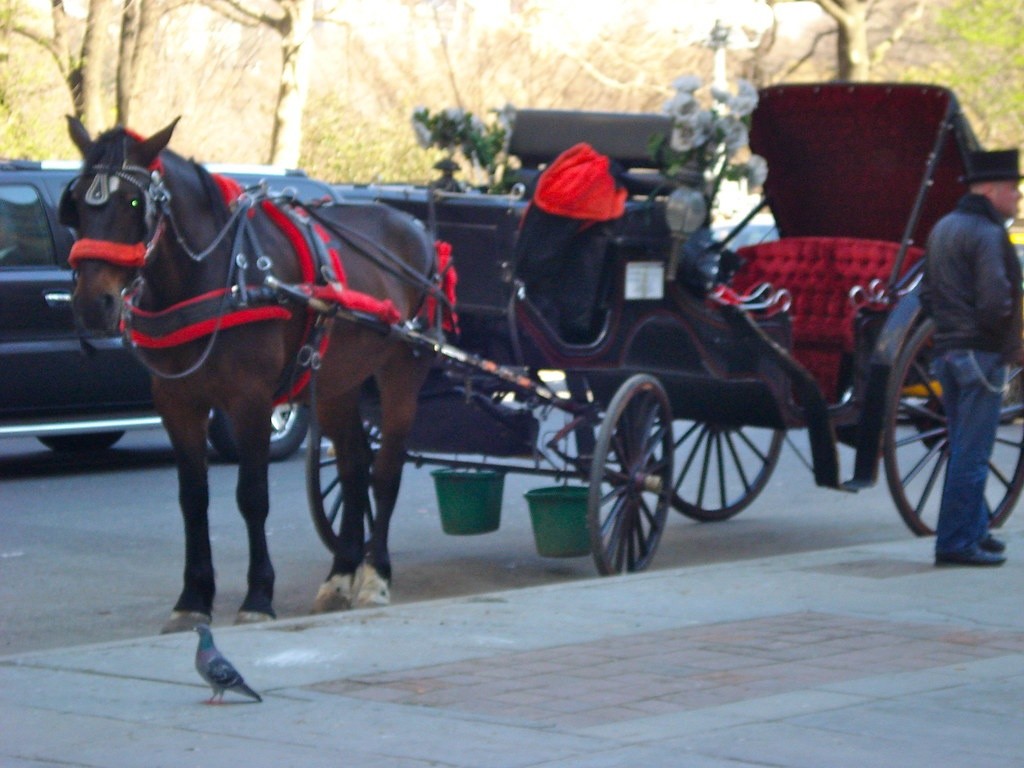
<box><xmin>919</xmin><ymin>149</ymin><xmax>1024</xmax><ymax>566</ymax></box>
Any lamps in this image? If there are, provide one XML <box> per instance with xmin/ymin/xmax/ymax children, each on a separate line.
<box><xmin>665</xmin><ymin>187</ymin><xmax>707</xmax><ymax>282</ymax></box>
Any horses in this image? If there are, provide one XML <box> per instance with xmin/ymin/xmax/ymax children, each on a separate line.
<box><xmin>57</xmin><ymin>113</ymin><xmax>438</xmax><ymax>636</ymax></box>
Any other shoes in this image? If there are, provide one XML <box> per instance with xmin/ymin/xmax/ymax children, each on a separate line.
<box><xmin>978</xmin><ymin>535</ymin><xmax>1004</xmax><ymax>553</ymax></box>
<box><xmin>935</xmin><ymin>542</ymin><xmax>1006</xmax><ymax>566</ymax></box>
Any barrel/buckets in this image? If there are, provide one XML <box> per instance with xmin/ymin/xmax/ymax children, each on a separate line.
<box><xmin>430</xmin><ymin>467</ymin><xmax>507</xmax><ymax>535</ymax></box>
<box><xmin>523</xmin><ymin>486</ymin><xmax>603</xmax><ymax>558</ymax></box>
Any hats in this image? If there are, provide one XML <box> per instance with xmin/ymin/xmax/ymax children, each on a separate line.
<box><xmin>965</xmin><ymin>149</ymin><xmax>1024</xmax><ymax>182</ymax></box>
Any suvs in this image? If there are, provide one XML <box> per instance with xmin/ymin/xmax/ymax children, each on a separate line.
<box><xmin>1</xmin><ymin>159</ymin><xmax>782</xmax><ymax>460</ymax></box>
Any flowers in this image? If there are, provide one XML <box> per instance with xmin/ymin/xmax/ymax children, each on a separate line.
<box><xmin>646</xmin><ymin>71</ymin><xmax>767</xmax><ymax>227</ymax></box>
<box><xmin>411</xmin><ymin>105</ymin><xmax>505</xmax><ymax>183</ymax></box>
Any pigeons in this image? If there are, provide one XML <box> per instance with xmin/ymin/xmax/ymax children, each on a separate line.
<box><xmin>191</xmin><ymin>621</ymin><xmax>263</xmax><ymax>705</ymax></box>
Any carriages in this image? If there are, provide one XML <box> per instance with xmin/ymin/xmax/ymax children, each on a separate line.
<box><xmin>58</xmin><ymin>81</ymin><xmax>1024</xmax><ymax>635</ymax></box>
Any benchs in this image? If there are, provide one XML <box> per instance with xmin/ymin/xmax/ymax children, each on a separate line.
<box><xmin>726</xmin><ymin>237</ymin><xmax>926</xmax><ymax>399</ymax></box>
<box><xmin>324</xmin><ymin>108</ymin><xmax>701</xmax><ymax>342</ymax></box>
